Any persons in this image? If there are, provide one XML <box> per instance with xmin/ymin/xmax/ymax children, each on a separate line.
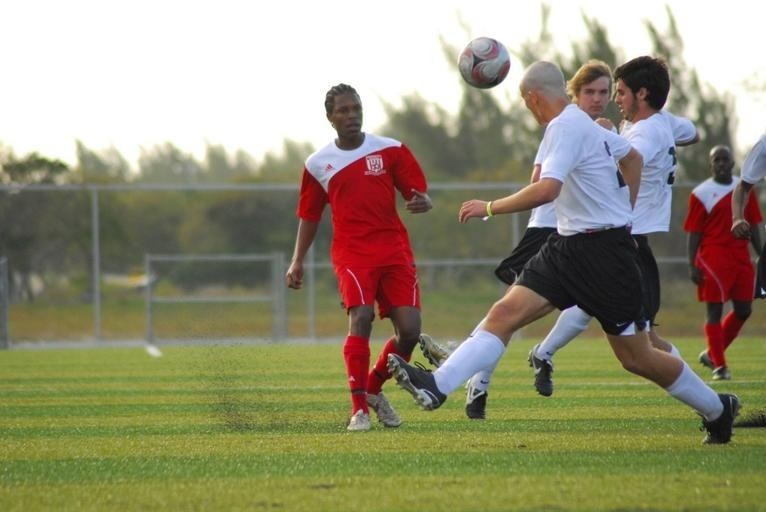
<box><xmin>729</xmin><ymin>132</ymin><xmax>765</xmax><ymax>300</ymax></box>
<box><xmin>416</xmin><ymin>53</ymin><xmax>701</xmax><ymax>373</ymax></box>
<box><xmin>384</xmin><ymin>61</ymin><xmax>742</xmax><ymax>445</ymax></box>
<box><xmin>284</xmin><ymin>83</ymin><xmax>437</xmax><ymax>434</ymax></box>
<box><xmin>465</xmin><ymin>58</ymin><xmax>617</xmax><ymax>423</ymax></box>
<box><xmin>682</xmin><ymin>144</ymin><xmax>764</xmax><ymax>380</ymax></box>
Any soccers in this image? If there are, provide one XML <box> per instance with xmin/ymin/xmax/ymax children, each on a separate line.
<box><xmin>458</xmin><ymin>37</ymin><xmax>509</xmax><ymax>90</ymax></box>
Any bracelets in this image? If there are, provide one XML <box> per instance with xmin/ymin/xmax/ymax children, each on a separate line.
<box><xmin>484</xmin><ymin>199</ymin><xmax>495</xmax><ymax>221</ymax></box>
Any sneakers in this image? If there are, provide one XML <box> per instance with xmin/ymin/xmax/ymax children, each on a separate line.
<box><xmin>699</xmin><ymin>350</ymin><xmax>731</xmax><ymax>380</ymax></box>
<box><xmin>528</xmin><ymin>344</ymin><xmax>553</xmax><ymax>396</ymax></box>
<box><xmin>347</xmin><ymin>333</ymin><xmax>487</xmax><ymax>431</ymax></box>
<box><xmin>696</xmin><ymin>394</ymin><xmax>742</xmax><ymax>444</ymax></box>
